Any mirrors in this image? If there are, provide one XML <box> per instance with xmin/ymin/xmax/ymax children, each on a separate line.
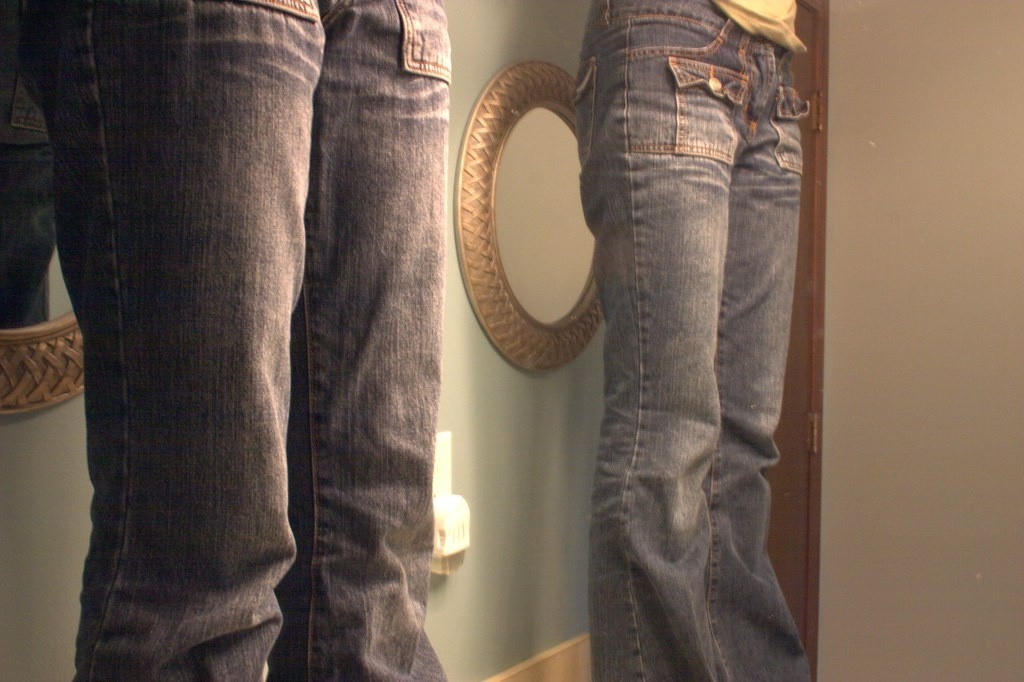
<box><xmin>454</xmin><ymin>59</ymin><xmax>606</xmax><ymax>373</ymax></box>
<box><xmin>0</xmin><ymin>0</ymin><xmax>74</xmax><ymax>416</ymax></box>
<box><xmin>435</xmin><ymin>0</ymin><xmax>1024</xmax><ymax>682</ymax></box>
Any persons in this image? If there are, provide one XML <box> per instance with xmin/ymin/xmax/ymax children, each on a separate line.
<box><xmin>28</xmin><ymin>0</ymin><xmax>453</xmax><ymax>682</ymax></box>
<box><xmin>570</xmin><ymin>0</ymin><xmax>811</xmax><ymax>682</ymax></box>
